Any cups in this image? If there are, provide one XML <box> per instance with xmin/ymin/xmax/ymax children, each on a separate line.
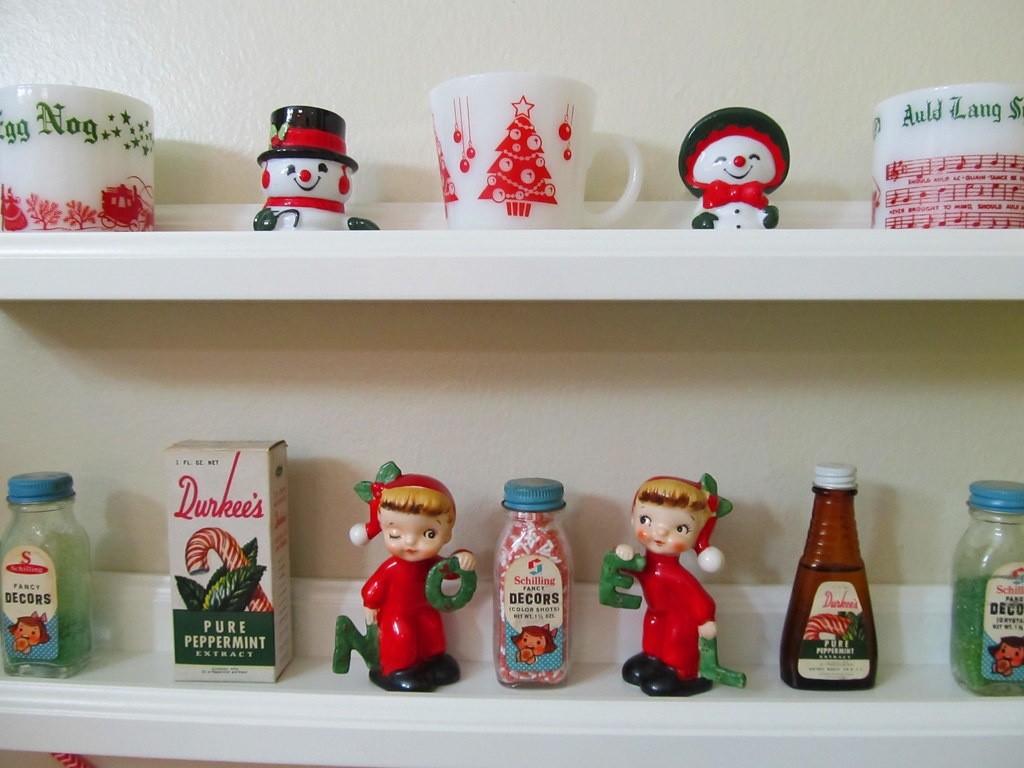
<box><xmin>0</xmin><ymin>81</ymin><xmax>156</xmax><ymax>233</ymax></box>
<box><xmin>430</xmin><ymin>72</ymin><xmax>645</xmax><ymax>229</ymax></box>
<box><xmin>871</xmin><ymin>82</ymin><xmax>1024</xmax><ymax>230</ymax></box>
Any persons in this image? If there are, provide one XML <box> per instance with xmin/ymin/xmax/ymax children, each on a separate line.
<box><xmin>349</xmin><ymin>460</ymin><xmax>476</xmax><ymax>692</ymax></box>
<box><xmin>614</xmin><ymin>474</ymin><xmax>731</xmax><ymax>695</ymax></box>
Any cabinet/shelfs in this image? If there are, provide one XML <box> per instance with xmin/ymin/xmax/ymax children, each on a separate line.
<box><xmin>0</xmin><ymin>202</ymin><xmax>1024</xmax><ymax>767</ymax></box>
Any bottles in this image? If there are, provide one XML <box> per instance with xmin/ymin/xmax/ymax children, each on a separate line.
<box><xmin>780</xmin><ymin>460</ymin><xmax>878</xmax><ymax>692</ymax></box>
<box><xmin>491</xmin><ymin>476</ymin><xmax>571</xmax><ymax>692</ymax></box>
<box><xmin>0</xmin><ymin>470</ymin><xmax>95</xmax><ymax>679</ymax></box>
<box><xmin>949</xmin><ymin>481</ymin><xmax>1024</xmax><ymax>698</ymax></box>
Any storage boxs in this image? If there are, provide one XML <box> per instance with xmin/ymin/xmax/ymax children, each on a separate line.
<box><xmin>168</xmin><ymin>439</ymin><xmax>293</xmax><ymax>684</ymax></box>
